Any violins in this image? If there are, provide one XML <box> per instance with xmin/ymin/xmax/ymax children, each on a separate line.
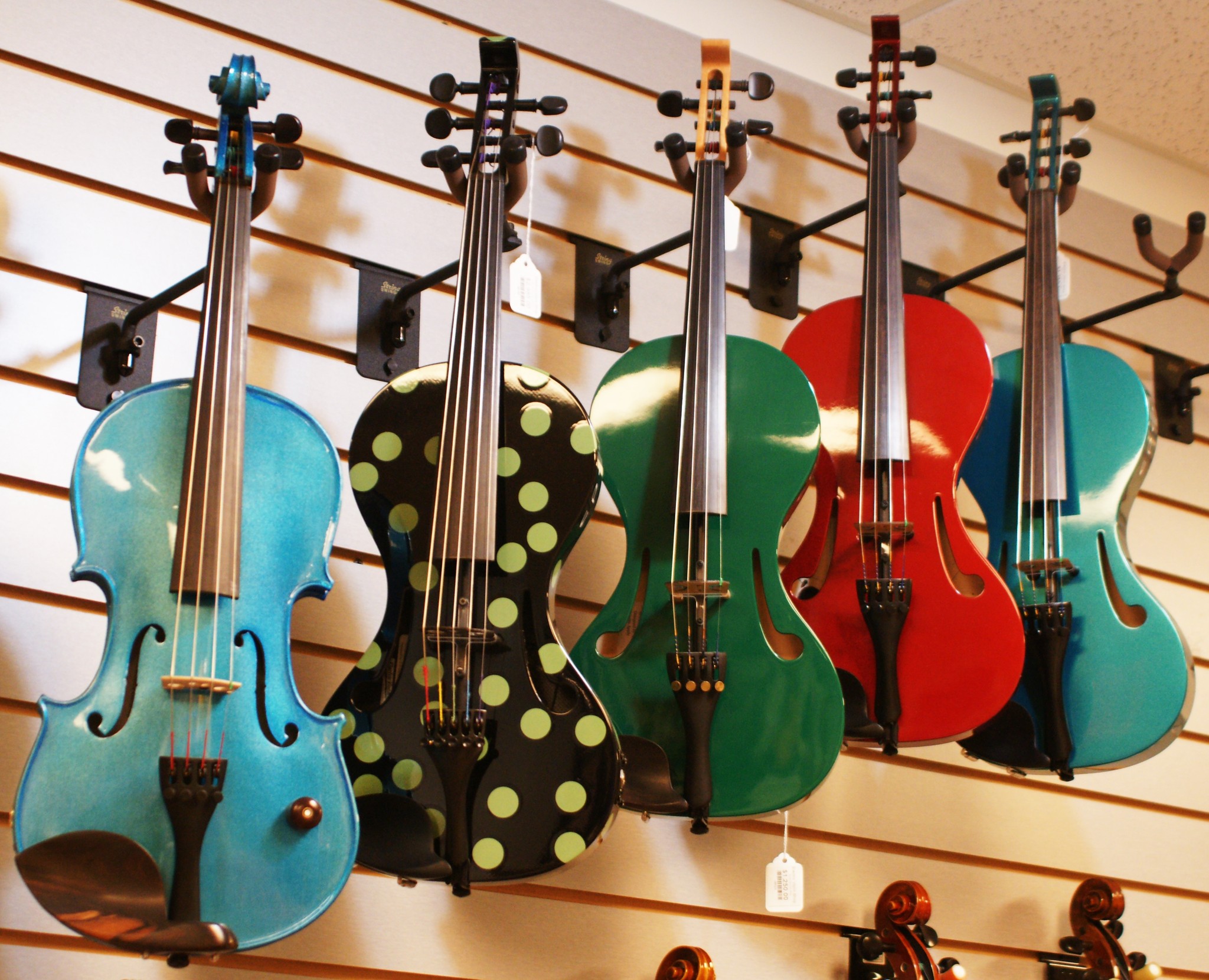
<box><xmin>654</xmin><ymin>945</ymin><xmax>716</xmax><ymax>980</ymax></box>
<box><xmin>566</xmin><ymin>40</ymin><xmax>845</xmax><ymax>835</ymax></box>
<box><xmin>951</xmin><ymin>74</ymin><xmax>1197</xmax><ymax>781</ymax></box>
<box><xmin>780</xmin><ymin>14</ymin><xmax>1027</xmax><ymax>757</ymax></box>
<box><xmin>856</xmin><ymin>880</ymin><xmax>966</xmax><ymax>980</ymax></box>
<box><xmin>11</xmin><ymin>53</ymin><xmax>360</xmax><ymax>968</ymax></box>
<box><xmin>322</xmin><ymin>33</ymin><xmax>629</xmax><ymax>896</ymax></box>
<box><xmin>1060</xmin><ymin>876</ymin><xmax>1162</xmax><ymax>980</ymax></box>
